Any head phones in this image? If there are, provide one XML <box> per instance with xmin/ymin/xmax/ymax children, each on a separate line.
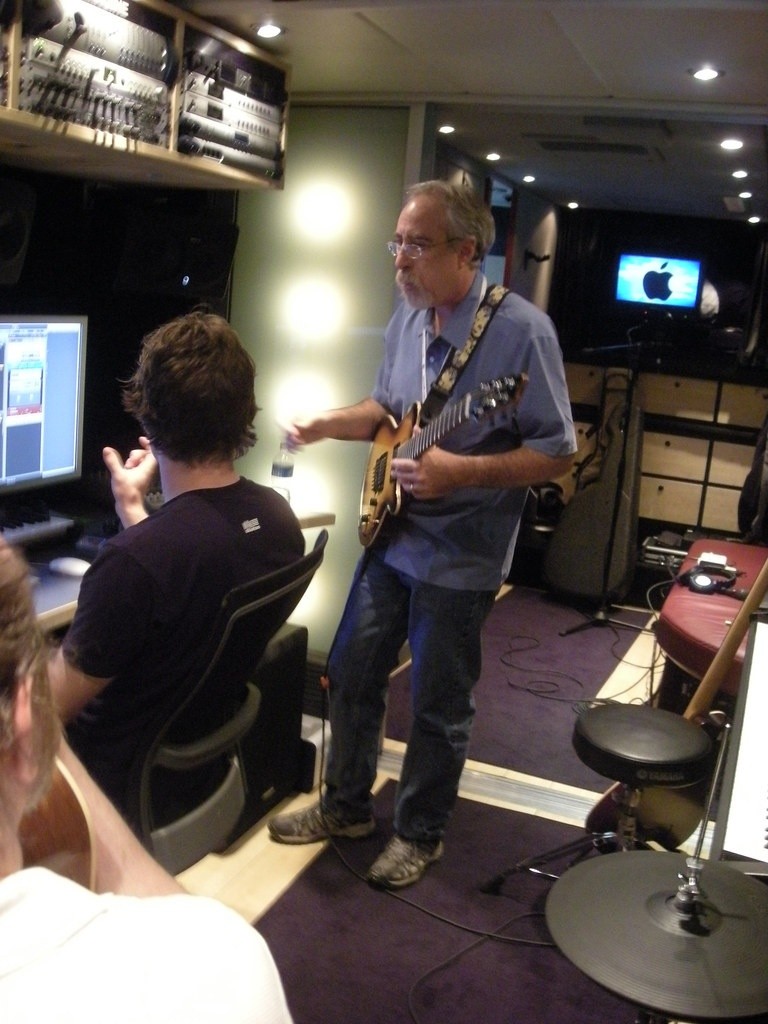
<box><xmin>676</xmin><ymin>565</ymin><xmax>737</xmax><ymax>594</ymax></box>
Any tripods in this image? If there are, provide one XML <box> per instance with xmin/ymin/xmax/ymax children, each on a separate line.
<box><xmin>559</xmin><ymin>311</ymin><xmax>652</xmax><ymax>636</ymax></box>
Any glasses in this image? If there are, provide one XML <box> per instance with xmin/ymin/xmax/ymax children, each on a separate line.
<box><xmin>387</xmin><ymin>235</ymin><xmax>467</xmax><ymax>258</ymax></box>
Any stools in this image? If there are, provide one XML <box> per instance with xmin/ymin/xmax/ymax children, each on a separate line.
<box><xmin>474</xmin><ymin>702</ymin><xmax>717</xmax><ymax>894</ymax></box>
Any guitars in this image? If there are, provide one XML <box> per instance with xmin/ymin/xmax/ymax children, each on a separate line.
<box><xmin>356</xmin><ymin>371</ymin><xmax>531</xmax><ymax>551</ymax></box>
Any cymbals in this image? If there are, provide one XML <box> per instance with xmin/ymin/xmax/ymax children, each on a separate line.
<box><xmin>542</xmin><ymin>851</ymin><xmax>768</xmax><ymax>1023</ymax></box>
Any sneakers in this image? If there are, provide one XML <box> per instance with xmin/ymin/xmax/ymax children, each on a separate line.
<box><xmin>268</xmin><ymin>802</ymin><xmax>377</xmax><ymax>844</ymax></box>
<box><xmin>366</xmin><ymin>834</ymin><xmax>443</xmax><ymax>891</ymax></box>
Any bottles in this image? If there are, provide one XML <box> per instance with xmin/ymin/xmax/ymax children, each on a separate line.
<box><xmin>273</xmin><ymin>443</ymin><xmax>293</xmax><ymax>505</ymax></box>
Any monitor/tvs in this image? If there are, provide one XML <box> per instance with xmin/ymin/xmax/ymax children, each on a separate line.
<box><xmin>613</xmin><ymin>254</ymin><xmax>705</xmax><ymax>318</ymax></box>
<box><xmin>0</xmin><ymin>314</ymin><xmax>88</xmax><ymax>495</ymax></box>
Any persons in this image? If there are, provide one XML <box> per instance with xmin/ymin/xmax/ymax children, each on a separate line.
<box><xmin>46</xmin><ymin>312</ymin><xmax>307</xmax><ymax>816</ymax></box>
<box><xmin>0</xmin><ymin>533</ymin><xmax>294</xmax><ymax>1024</ymax></box>
<box><xmin>738</xmin><ymin>409</ymin><xmax>768</xmax><ymax>547</ymax></box>
<box><xmin>281</xmin><ymin>178</ymin><xmax>577</xmax><ymax>892</ymax></box>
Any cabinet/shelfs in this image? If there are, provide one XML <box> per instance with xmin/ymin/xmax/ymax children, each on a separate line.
<box><xmin>0</xmin><ymin>0</ymin><xmax>290</xmax><ymax>192</ymax></box>
<box><xmin>547</xmin><ymin>358</ymin><xmax>768</xmax><ymax>539</ymax></box>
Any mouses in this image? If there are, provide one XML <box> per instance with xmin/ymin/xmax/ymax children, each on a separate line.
<box><xmin>48</xmin><ymin>557</ymin><xmax>91</xmax><ymax>576</ymax></box>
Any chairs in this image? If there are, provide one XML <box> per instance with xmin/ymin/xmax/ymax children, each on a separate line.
<box><xmin>136</xmin><ymin>528</ymin><xmax>330</xmax><ymax>876</ymax></box>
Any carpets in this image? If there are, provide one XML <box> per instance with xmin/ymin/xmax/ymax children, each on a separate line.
<box><xmin>387</xmin><ymin>588</ymin><xmax>655</xmax><ymax>792</ymax></box>
<box><xmin>252</xmin><ymin>778</ymin><xmax>768</xmax><ymax>1024</ymax></box>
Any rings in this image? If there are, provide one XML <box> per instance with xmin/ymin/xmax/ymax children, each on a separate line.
<box><xmin>410</xmin><ymin>483</ymin><xmax>413</xmax><ymax>490</ymax></box>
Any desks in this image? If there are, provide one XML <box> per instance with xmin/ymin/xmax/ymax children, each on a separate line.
<box><xmin>654</xmin><ymin>538</ymin><xmax>768</xmax><ymax>695</ymax></box>
<box><xmin>30</xmin><ymin>556</ymin><xmax>101</xmax><ymax>630</ymax></box>
